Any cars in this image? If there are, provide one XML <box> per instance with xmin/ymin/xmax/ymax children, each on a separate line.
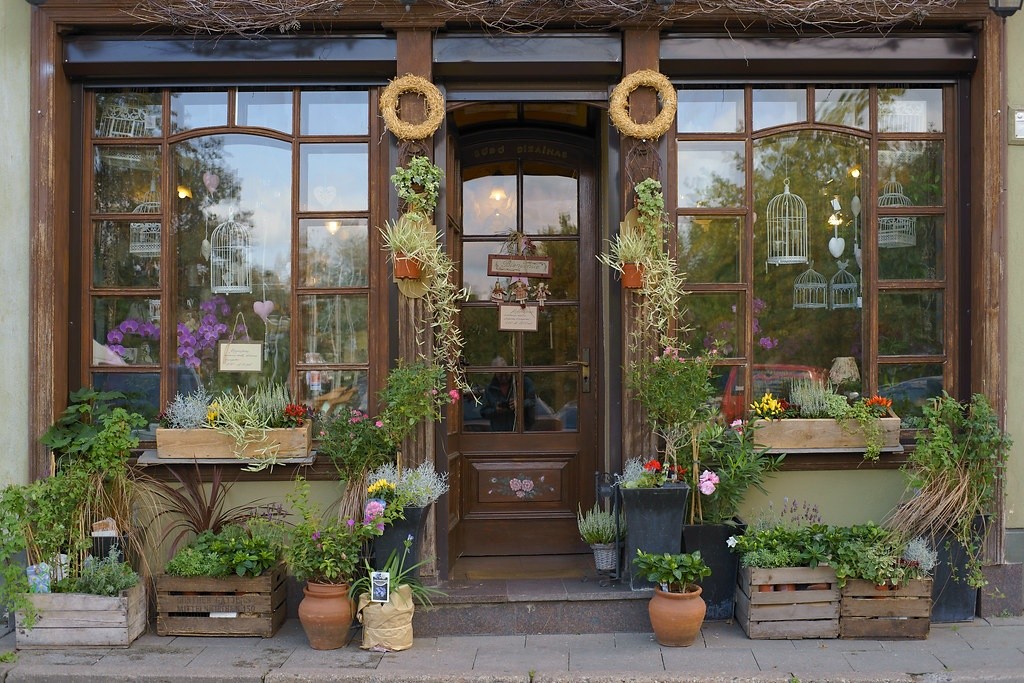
<box><xmin>555</xmin><ymin>400</ymin><xmax>579</xmax><ymax>429</ymax></box>
<box><xmin>90</xmin><ymin>363</ymin><xmax>206</xmax><ymax>442</ymax></box>
<box><xmin>847</xmin><ymin>376</ymin><xmax>944</xmax><ymax>419</ymax></box>
<box><xmin>325</xmin><ymin>376</ymin><xmax>369</xmax><ymax>420</ymax></box>
<box><xmin>461</xmin><ymin>388</ymin><xmax>555</xmax><ymax>432</ymax></box>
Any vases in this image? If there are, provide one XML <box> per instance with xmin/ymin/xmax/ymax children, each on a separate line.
<box><xmin>298</xmin><ymin>579</ymin><xmax>356</xmax><ymax>650</ymax></box>
<box><xmin>156</xmin><ymin>419</ymin><xmax>312</xmax><ymax>460</ymax></box>
<box><xmin>753</xmin><ymin>409</ymin><xmax>902</xmax><ymax>446</ymax></box>
<box><xmin>735</xmin><ymin>561</ymin><xmax>841</xmax><ymax>640</ymax></box>
<box><xmin>682</xmin><ymin>515</ymin><xmax>748</xmax><ymax>621</ymax></box>
<box><xmin>374</xmin><ymin>504</ymin><xmax>431</xmax><ymax>579</ymax></box>
<box><xmin>619</xmin><ymin>483</ymin><xmax>690</xmax><ymax>588</ymax></box>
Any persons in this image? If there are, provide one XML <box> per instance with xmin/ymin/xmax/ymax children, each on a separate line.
<box><xmin>480</xmin><ymin>372</ymin><xmax>536</xmax><ymax>432</ymax></box>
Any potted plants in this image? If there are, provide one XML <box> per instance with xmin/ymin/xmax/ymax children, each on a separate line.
<box><xmin>0</xmin><ymin>386</ymin><xmax>148</xmax><ymax>650</ymax></box>
<box><xmin>376</xmin><ymin>154</ymin><xmax>481</xmax><ymax>407</ymax></box>
<box><xmin>633</xmin><ymin>548</ymin><xmax>712</xmax><ymax>647</ymax></box>
<box><xmin>595</xmin><ymin>177</ymin><xmax>696</xmax><ymax>355</ymax></box>
<box><xmin>834</xmin><ymin>390</ymin><xmax>1015</xmax><ymax>639</ymax></box>
<box><xmin>154</xmin><ymin>507</ymin><xmax>288</xmax><ymax>639</ymax></box>
<box><xmin>577</xmin><ymin>502</ymin><xmax>626</xmax><ymax>569</ymax></box>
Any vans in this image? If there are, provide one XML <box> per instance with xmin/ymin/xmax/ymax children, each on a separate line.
<box><xmin>719</xmin><ymin>363</ymin><xmax>831</xmax><ymax>428</ymax></box>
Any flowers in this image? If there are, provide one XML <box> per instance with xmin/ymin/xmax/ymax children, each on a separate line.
<box><xmin>610</xmin><ymin>296</ymin><xmax>893</xmax><ymax>570</ymax></box>
<box><xmin>108</xmin><ymin>295</ymin><xmax>462</xmax><ymax>620</ymax></box>
